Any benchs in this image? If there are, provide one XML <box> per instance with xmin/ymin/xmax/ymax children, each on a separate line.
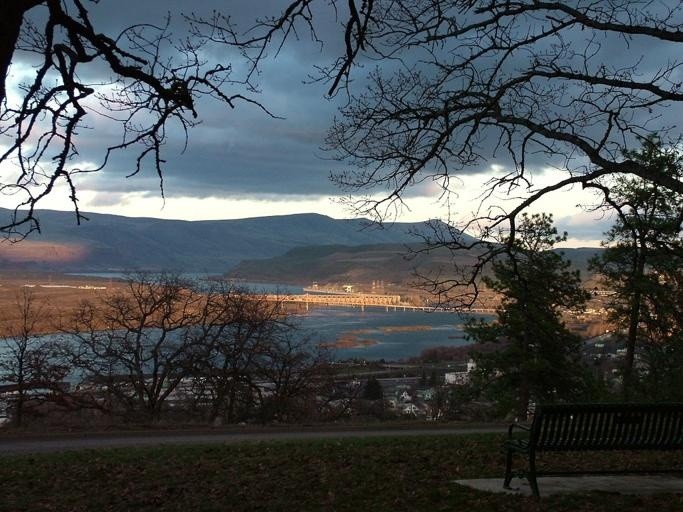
<box><xmin>507</xmin><ymin>398</ymin><xmax>536</xmax><ymax>437</ymax></box>
<box><xmin>503</xmin><ymin>399</ymin><xmax>683</xmax><ymax>492</ymax></box>
